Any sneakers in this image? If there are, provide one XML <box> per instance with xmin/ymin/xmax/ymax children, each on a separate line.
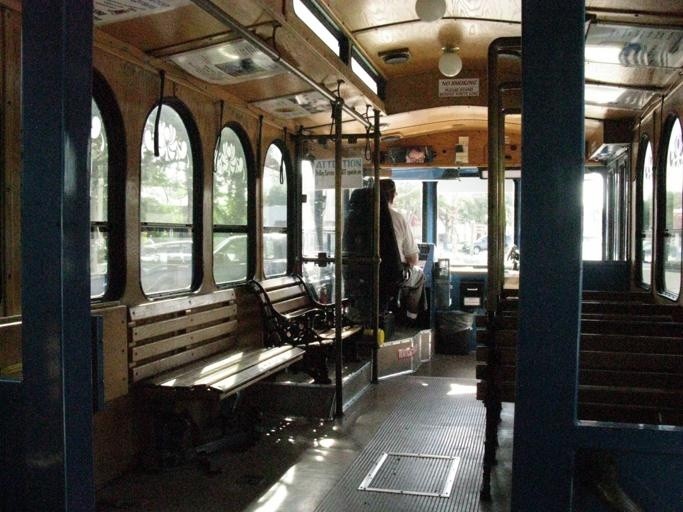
<box><xmin>407</xmin><ymin>316</ymin><xmax>418</xmax><ymax>324</ymax></box>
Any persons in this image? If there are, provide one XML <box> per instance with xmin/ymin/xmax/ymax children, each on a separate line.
<box><xmin>378</xmin><ymin>178</ymin><xmax>428</xmax><ymax>329</ymax></box>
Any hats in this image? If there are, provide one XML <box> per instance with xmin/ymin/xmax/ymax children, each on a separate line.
<box><xmin>380</xmin><ymin>179</ymin><xmax>397</xmax><ymax>195</ymax></box>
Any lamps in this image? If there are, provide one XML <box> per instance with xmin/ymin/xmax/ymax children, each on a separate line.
<box><xmin>412</xmin><ymin>0</ymin><xmax>448</xmax><ymax>23</ymax></box>
<box><xmin>435</xmin><ymin>40</ymin><xmax>465</xmax><ymax>79</ymax></box>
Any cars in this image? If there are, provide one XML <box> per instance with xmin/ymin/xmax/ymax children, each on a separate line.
<box><xmin>90</xmin><ymin>234</ymin><xmax>293</xmax><ymax>295</ymax></box>
<box><xmin>465</xmin><ymin>233</ymin><xmax>512</xmax><ymax>254</ymax></box>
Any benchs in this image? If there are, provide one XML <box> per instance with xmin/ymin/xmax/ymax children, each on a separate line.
<box><xmin>122</xmin><ymin>287</ymin><xmax>308</xmax><ymax>476</ymax></box>
<box><xmin>250</xmin><ymin>270</ymin><xmax>362</xmax><ymax>381</ymax></box>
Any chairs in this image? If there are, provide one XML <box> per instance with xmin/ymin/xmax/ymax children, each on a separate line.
<box><xmin>342</xmin><ymin>188</ymin><xmax>412</xmax><ymax>314</ymax></box>
